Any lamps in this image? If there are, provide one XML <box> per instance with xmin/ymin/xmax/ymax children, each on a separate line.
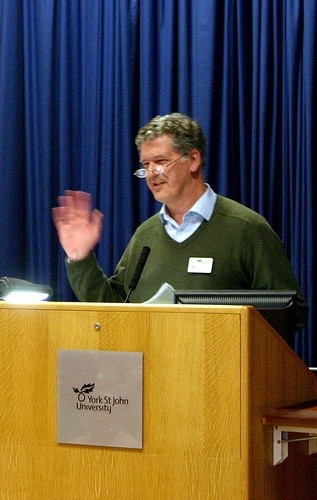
<box><xmin>0</xmin><ymin>276</ymin><xmax>54</xmax><ymax>301</ymax></box>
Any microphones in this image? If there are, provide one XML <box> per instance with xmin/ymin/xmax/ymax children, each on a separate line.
<box><xmin>124</xmin><ymin>246</ymin><xmax>150</xmax><ymax>303</ymax></box>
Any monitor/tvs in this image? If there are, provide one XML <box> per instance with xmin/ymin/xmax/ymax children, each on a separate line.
<box><xmin>173</xmin><ymin>289</ymin><xmax>296</xmax><ymax>352</ymax></box>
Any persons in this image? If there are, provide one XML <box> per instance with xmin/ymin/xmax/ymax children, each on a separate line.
<box><xmin>52</xmin><ymin>113</ymin><xmax>309</xmax><ymax>346</ymax></box>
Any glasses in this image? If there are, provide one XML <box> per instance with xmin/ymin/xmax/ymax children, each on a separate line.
<box><xmin>134</xmin><ymin>153</ymin><xmax>189</xmax><ymax>178</ymax></box>
